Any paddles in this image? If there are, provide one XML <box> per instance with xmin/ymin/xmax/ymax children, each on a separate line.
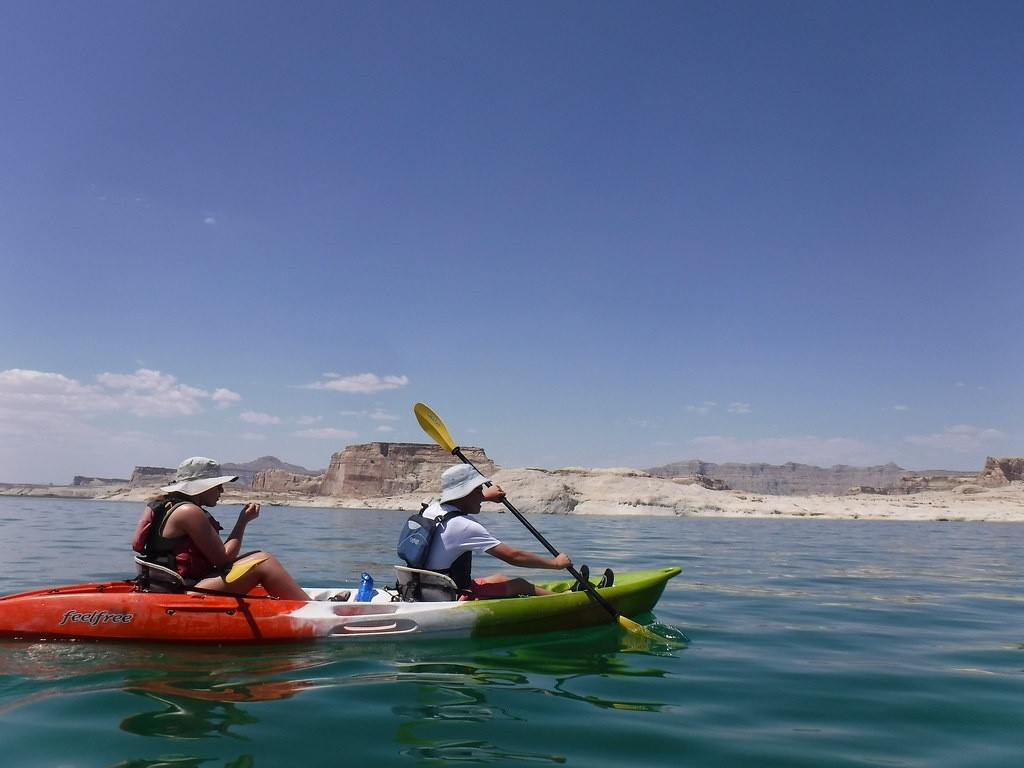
<box><xmin>412</xmin><ymin>400</ymin><xmax>643</xmax><ymax>634</ymax></box>
<box><xmin>225</xmin><ymin>550</ymin><xmax>269</xmax><ymax>583</ymax></box>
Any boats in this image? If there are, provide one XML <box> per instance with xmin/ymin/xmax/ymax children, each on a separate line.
<box><xmin>0</xmin><ymin>565</ymin><xmax>686</xmax><ymax>644</ymax></box>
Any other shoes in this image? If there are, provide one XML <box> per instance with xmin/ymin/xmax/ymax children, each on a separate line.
<box><xmin>597</xmin><ymin>568</ymin><xmax>614</xmax><ymax>588</ymax></box>
<box><xmin>569</xmin><ymin>564</ymin><xmax>590</xmax><ymax>592</ymax></box>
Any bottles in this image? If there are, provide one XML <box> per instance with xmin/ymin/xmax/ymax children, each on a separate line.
<box><xmin>356</xmin><ymin>572</ymin><xmax>373</xmax><ymax>602</ymax></box>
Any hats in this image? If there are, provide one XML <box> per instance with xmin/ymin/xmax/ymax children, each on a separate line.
<box><xmin>440</xmin><ymin>463</ymin><xmax>491</xmax><ymax>505</ymax></box>
<box><xmin>159</xmin><ymin>457</ymin><xmax>240</xmax><ymax>496</ymax></box>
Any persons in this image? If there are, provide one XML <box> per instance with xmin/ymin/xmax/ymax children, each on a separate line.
<box><xmin>396</xmin><ymin>462</ymin><xmax>614</xmax><ymax>600</ymax></box>
<box><xmin>132</xmin><ymin>456</ymin><xmax>350</xmax><ymax>603</ymax></box>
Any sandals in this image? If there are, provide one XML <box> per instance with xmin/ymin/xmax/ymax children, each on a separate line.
<box><xmin>328</xmin><ymin>591</ymin><xmax>350</xmax><ymax>602</ymax></box>
